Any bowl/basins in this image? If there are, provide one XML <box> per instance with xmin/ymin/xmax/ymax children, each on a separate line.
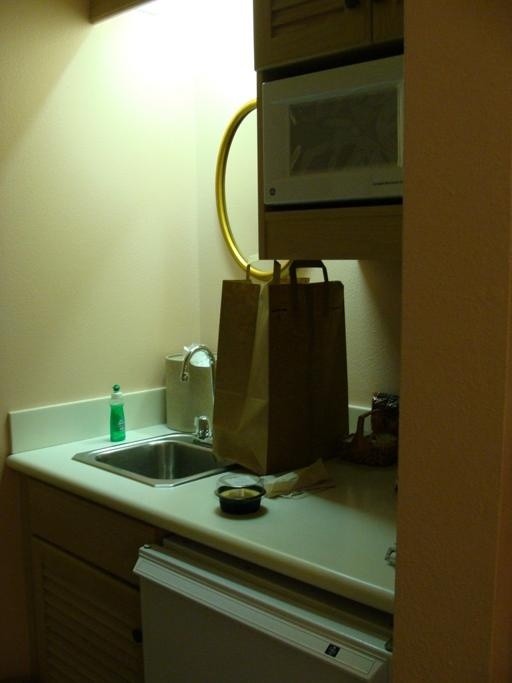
<box><xmin>216</xmin><ymin>484</ymin><xmax>268</xmax><ymax>514</ymax></box>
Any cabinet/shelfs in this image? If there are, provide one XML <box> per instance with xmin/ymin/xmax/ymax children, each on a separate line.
<box><xmin>9</xmin><ymin>469</ymin><xmax>160</xmax><ymax>682</ymax></box>
<box><xmin>252</xmin><ymin>0</ymin><xmax>407</xmax><ymax>72</ymax></box>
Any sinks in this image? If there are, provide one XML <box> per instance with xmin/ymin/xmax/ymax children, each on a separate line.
<box><xmin>72</xmin><ymin>436</ymin><xmax>227</xmax><ymax>489</ymax></box>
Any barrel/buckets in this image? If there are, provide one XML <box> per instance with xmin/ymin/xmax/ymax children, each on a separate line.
<box><xmin>164</xmin><ymin>353</ymin><xmax>218</xmax><ymax>434</ymax></box>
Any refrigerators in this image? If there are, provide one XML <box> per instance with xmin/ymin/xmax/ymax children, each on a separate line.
<box><xmin>132</xmin><ymin>540</ymin><xmax>399</xmax><ymax>683</ymax></box>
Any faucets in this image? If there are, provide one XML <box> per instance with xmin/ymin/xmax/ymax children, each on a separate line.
<box><xmin>181</xmin><ymin>345</ymin><xmax>216</xmax><ymax>407</ymax></box>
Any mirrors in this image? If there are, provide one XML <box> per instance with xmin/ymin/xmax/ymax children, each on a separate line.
<box><xmin>215</xmin><ymin>97</ymin><xmax>295</xmax><ymax>280</ymax></box>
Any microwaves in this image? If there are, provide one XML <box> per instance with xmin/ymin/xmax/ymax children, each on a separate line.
<box><xmin>261</xmin><ymin>58</ymin><xmax>406</xmax><ymax>210</ymax></box>
